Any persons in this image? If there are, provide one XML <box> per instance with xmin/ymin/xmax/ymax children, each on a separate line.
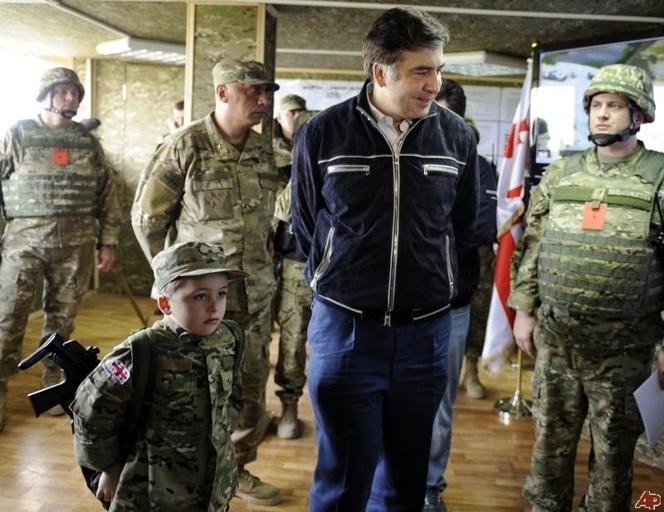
<box><xmin>270</xmin><ymin>105</ymin><xmax>327</xmax><ymax>442</ymax></box>
<box><xmin>457</xmin><ymin>113</ymin><xmax>502</xmax><ymax>401</ymax></box>
<box><xmin>157</xmin><ymin>100</ymin><xmax>187</xmax><ymax>148</ymax></box>
<box><xmin>503</xmin><ymin>59</ymin><xmax>664</xmax><ymax>512</ymax></box>
<box><xmin>275</xmin><ymin>94</ymin><xmax>309</xmax><ymax>157</ymax></box>
<box><xmin>424</xmin><ymin>77</ymin><xmax>484</xmax><ymax>512</ymax></box>
<box><xmin>126</xmin><ymin>53</ymin><xmax>283</xmax><ymax>505</ymax></box>
<box><xmin>0</xmin><ymin>66</ymin><xmax>127</xmax><ymax>435</ymax></box>
<box><xmin>69</xmin><ymin>240</ymin><xmax>252</xmax><ymax>512</ymax></box>
<box><xmin>292</xmin><ymin>1</ymin><xmax>483</xmax><ymax>512</ymax></box>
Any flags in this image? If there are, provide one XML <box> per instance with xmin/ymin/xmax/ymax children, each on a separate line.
<box><xmin>477</xmin><ymin>57</ymin><xmax>538</xmax><ymax>380</ymax></box>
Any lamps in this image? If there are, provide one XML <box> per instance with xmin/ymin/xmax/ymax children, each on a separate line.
<box><xmin>96</xmin><ymin>36</ymin><xmax>186</xmax><ymax>66</ymax></box>
<box><xmin>441</xmin><ymin>50</ymin><xmax>528</xmax><ymax>77</ymax></box>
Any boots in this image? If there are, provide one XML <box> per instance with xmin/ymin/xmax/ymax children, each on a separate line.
<box><xmin>461</xmin><ymin>357</ymin><xmax>485</xmax><ymax>399</ymax></box>
<box><xmin>275</xmin><ymin>391</ymin><xmax>301</xmax><ymax>439</ymax></box>
<box><xmin>41</xmin><ymin>367</ymin><xmax>66</xmax><ymax>416</ymax></box>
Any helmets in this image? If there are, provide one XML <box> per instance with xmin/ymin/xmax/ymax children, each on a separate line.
<box><xmin>37</xmin><ymin>67</ymin><xmax>84</xmax><ymax>103</ymax></box>
<box><xmin>583</xmin><ymin>64</ymin><xmax>655</xmax><ymax>122</ymax></box>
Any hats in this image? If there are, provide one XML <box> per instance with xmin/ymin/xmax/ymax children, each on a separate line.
<box><xmin>212</xmin><ymin>59</ymin><xmax>279</xmax><ymax>91</ymax></box>
<box><xmin>151</xmin><ymin>242</ymin><xmax>249</xmax><ymax>289</ymax></box>
<box><xmin>281</xmin><ymin>95</ymin><xmax>306</xmax><ymax>110</ymax></box>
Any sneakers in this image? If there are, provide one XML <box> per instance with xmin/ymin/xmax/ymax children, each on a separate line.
<box><xmin>422</xmin><ymin>488</ymin><xmax>446</xmax><ymax>512</ymax></box>
<box><xmin>235</xmin><ymin>470</ymin><xmax>280</xmax><ymax>505</ymax></box>
